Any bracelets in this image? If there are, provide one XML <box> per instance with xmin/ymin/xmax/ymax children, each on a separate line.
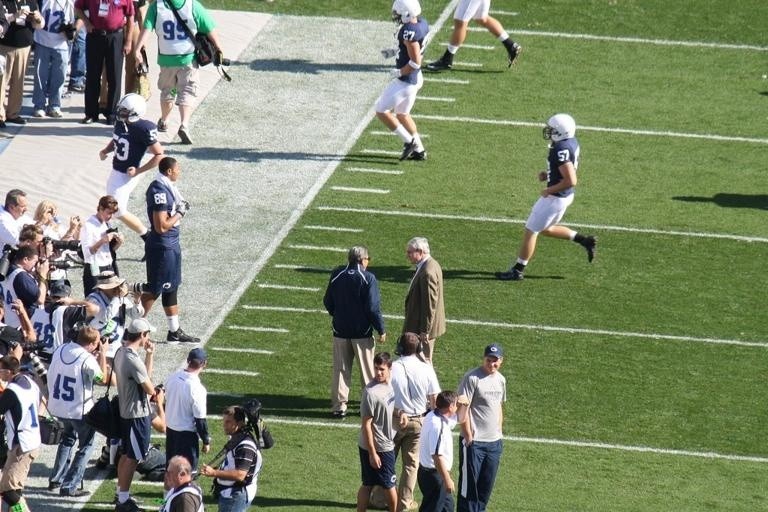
<box><xmin>398</xmin><ymin>409</ymin><xmax>404</xmax><ymax>416</ymax></box>
<box><xmin>463</xmin><ymin>403</ymin><xmax>469</xmax><ymax>406</ymax></box>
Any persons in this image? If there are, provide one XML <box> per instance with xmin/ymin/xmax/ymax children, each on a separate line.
<box><xmin>375</xmin><ymin>0</ymin><xmax>428</xmax><ymax>161</ymax></box>
<box><xmin>457</xmin><ymin>344</ymin><xmax>507</xmax><ymax>512</ymax></box>
<box><xmin>2</xmin><ymin>189</ymin><xmax>261</xmax><ymax>511</ymax></box>
<box><xmin>141</xmin><ymin>157</ymin><xmax>201</xmax><ymax>346</ymax></box>
<box><xmin>324</xmin><ymin>246</ymin><xmax>386</xmax><ymax>419</ymax></box>
<box><xmin>426</xmin><ymin>0</ymin><xmax>522</xmax><ymax>71</ymax></box>
<box><xmin>357</xmin><ymin>351</ymin><xmax>408</xmax><ymax>512</ymax></box>
<box><xmin>1</xmin><ymin>0</ymin><xmax>223</xmax><ymax>144</ymax></box>
<box><xmin>402</xmin><ymin>236</ymin><xmax>447</xmax><ymax>416</ymax></box>
<box><xmin>98</xmin><ymin>93</ymin><xmax>163</xmax><ymax>263</ymax></box>
<box><xmin>417</xmin><ymin>390</ymin><xmax>469</xmax><ymax>512</ymax></box>
<box><xmin>494</xmin><ymin>112</ymin><xmax>598</xmax><ymax>281</ymax></box>
<box><xmin>369</xmin><ymin>332</ymin><xmax>442</xmax><ymax>512</ymax></box>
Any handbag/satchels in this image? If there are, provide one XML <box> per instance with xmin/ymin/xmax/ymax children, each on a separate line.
<box><xmin>85</xmin><ymin>395</ymin><xmax>121</xmax><ymax>439</ymax></box>
<box><xmin>195</xmin><ymin>32</ymin><xmax>214</xmax><ymax>65</ymax></box>
<box><xmin>39</xmin><ymin>418</ymin><xmax>64</xmax><ymax>445</ymax></box>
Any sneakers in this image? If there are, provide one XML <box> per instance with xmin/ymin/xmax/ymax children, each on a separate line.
<box><xmin>425</xmin><ymin>59</ymin><xmax>453</xmax><ymax>71</ymax></box>
<box><xmin>0</xmin><ymin>86</ymin><xmax>116</xmax><ymax>128</ymax></box>
<box><xmin>61</xmin><ymin>489</ymin><xmax>90</xmax><ymax>497</ymax></box>
<box><xmin>178</xmin><ymin>127</ymin><xmax>193</xmax><ymax>145</ymax></box>
<box><xmin>508</xmin><ymin>44</ymin><xmax>521</xmax><ymax>68</ymax></box>
<box><xmin>166</xmin><ymin>327</ymin><xmax>200</xmax><ymax>344</ymax></box>
<box><xmin>49</xmin><ymin>483</ymin><xmax>61</xmax><ymax>491</ymax></box>
<box><xmin>114</xmin><ymin>493</ymin><xmax>145</xmax><ymax>511</ymax></box>
<box><xmin>494</xmin><ymin>268</ymin><xmax>524</xmax><ymax>282</ymax></box>
<box><xmin>157</xmin><ymin>117</ymin><xmax>167</xmax><ymax>132</ymax></box>
<box><xmin>585</xmin><ymin>234</ymin><xmax>596</xmax><ymax>262</ymax></box>
<box><xmin>398</xmin><ymin>137</ymin><xmax>428</xmax><ymax>162</ymax></box>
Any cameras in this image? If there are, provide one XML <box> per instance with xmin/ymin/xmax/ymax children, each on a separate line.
<box><xmin>73</xmin><ymin>216</ymin><xmax>80</xmax><ymax>222</ymax></box>
<box><xmin>95</xmin><ymin>331</ymin><xmax>119</xmax><ymax>351</ymax></box>
<box><xmin>43</xmin><ymin>237</ymin><xmax>80</xmax><ymax>251</ymax></box>
<box><xmin>213</xmin><ymin>51</ymin><xmax>230</xmax><ymax>66</ymax></box>
<box><xmin>63</xmin><ymin>24</ymin><xmax>77</xmax><ymax>41</ymax></box>
<box><xmin>120</xmin><ymin>279</ymin><xmax>152</xmax><ymax>298</ymax></box>
<box><xmin>150</xmin><ymin>383</ymin><xmax>166</xmax><ymax>402</ymax></box>
<box><xmin>8</xmin><ymin>341</ymin><xmax>46</xmax><ymax>352</ymax></box>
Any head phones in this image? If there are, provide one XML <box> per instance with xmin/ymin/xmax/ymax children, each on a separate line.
<box><xmin>397</xmin><ymin>332</ymin><xmax>424</xmax><ymax>353</ymax></box>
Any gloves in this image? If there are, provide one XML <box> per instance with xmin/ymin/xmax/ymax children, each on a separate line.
<box><xmin>390</xmin><ymin>68</ymin><xmax>402</xmax><ymax>79</ymax></box>
<box><xmin>382</xmin><ymin>48</ymin><xmax>397</xmax><ymax>58</ymax></box>
<box><xmin>178</xmin><ymin>201</ymin><xmax>190</xmax><ymax>218</ymax></box>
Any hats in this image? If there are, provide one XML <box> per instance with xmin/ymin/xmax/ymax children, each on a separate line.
<box><xmin>189</xmin><ymin>348</ymin><xmax>206</xmax><ymax>362</ymax></box>
<box><xmin>128</xmin><ymin>317</ymin><xmax>158</xmax><ymax>333</ymax></box>
<box><xmin>485</xmin><ymin>344</ymin><xmax>503</xmax><ymax>358</ymax></box>
<box><xmin>51</xmin><ymin>279</ymin><xmax>70</xmax><ymax>297</ymax></box>
<box><xmin>92</xmin><ymin>271</ymin><xmax>125</xmax><ymax>291</ymax></box>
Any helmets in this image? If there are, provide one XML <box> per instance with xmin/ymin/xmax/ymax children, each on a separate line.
<box><xmin>115</xmin><ymin>94</ymin><xmax>147</xmax><ymax>121</ymax></box>
<box><xmin>543</xmin><ymin>112</ymin><xmax>577</xmax><ymax>141</ymax></box>
<box><xmin>391</xmin><ymin>0</ymin><xmax>422</xmax><ymax>25</ymax></box>
